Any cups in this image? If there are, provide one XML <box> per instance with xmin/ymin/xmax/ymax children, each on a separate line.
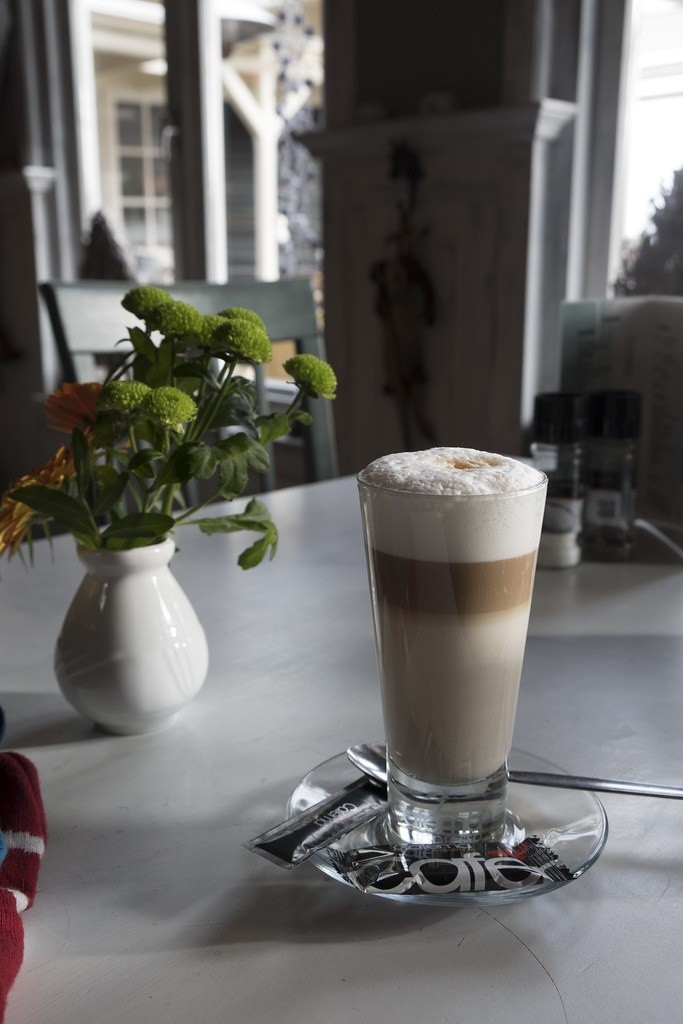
<box><xmin>288</xmin><ymin>457</ymin><xmax>609</xmax><ymax>847</ymax></box>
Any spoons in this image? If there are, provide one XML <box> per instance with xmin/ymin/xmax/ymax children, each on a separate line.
<box><xmin>343</xmin><ymin>740</ymin><xmax>683</xmax><ymax>802</ymax></box>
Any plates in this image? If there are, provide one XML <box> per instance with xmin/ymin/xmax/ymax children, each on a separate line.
<box><xmin>289</xmin><ymin>737</ymin><xmax>609</xmax><ymax>904</ymax></box>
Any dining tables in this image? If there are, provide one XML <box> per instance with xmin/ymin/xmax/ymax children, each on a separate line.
<box><xmin>0</xmin><ymin>430</ymin><xmax>683</xmax><ymax>1024</ymax></box>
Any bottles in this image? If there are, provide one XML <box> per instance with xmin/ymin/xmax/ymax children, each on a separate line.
<box><xmin>530</xmin><ymin>390</ymin><xmax>583</xmax><ymax>569</ymax></box>
<box><xmin>588</xmin><ymin>390</ymin><xmax>642</xmax><ymax>562</ymax></box>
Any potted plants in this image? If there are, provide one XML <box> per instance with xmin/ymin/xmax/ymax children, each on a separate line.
<box><xmin>0</xmin><ymin>286</ymin><xmax>339</xmax><ymax>747</ymax></box>
